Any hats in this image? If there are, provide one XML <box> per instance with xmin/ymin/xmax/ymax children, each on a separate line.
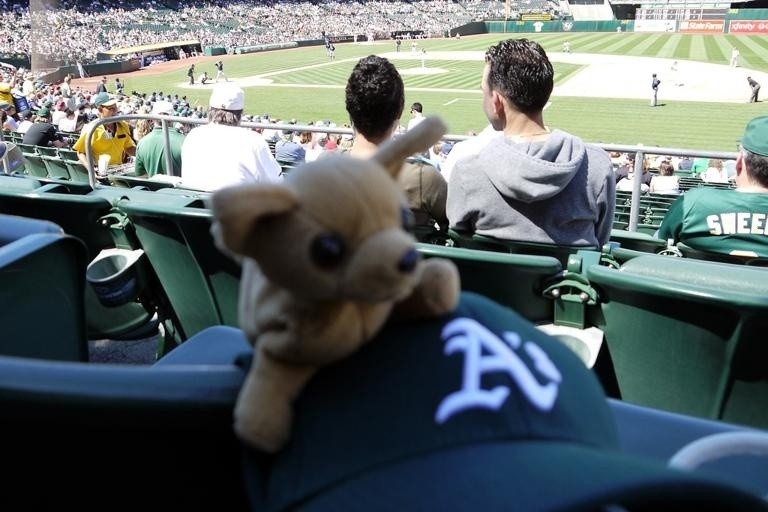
<box><xmin>78</xmin><ymin>104</ymin><xmax>86</xmax><ymax>111</ymax></box>
<box><xmin>38</xmin><ymin>108</ymin><xmax>50</xmax><ymax>117</ymax></box>
<box><xmin>94</xmin><ymin>93</ymin><xmax>117</xmax><ymax>108</ymax></box>
<box><xmin>441</xmin><ymin>143</ymin><xmax>454</xmax><ymax>155</ymax></box>
<box><xmin>100</xmin><ymin>76</ymin><xmax>107</xmax><ymax>81</ymax></box>
<box><xmin>209</xmin><ymin>84</ymin><xmax>245</xmax><ymax>112</ymax></box>
<box><xmin>57</xmin><ymin>101</ymin><xmax>66</xmax><ymax>111</ymax></box>
<box><xmin>131</xmin><ymin>91</ymin><xmax>353</xmax><ymax>142</ymax></box>
<box><xmin>741</xmin><ymin>115</ymin><xmax>768</xmax><ymax>156</ymax></box>
<box><xmin>22</xmin><ymin>110</ymin><xmax>33</xmax><ymax>120</ymax></box>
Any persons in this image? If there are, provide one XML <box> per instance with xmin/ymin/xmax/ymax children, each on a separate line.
<box><xmin>317</xmin><ymin>54</ymin><xmax>449</xmax><ymax>231</ymax></box>
<box><xmin>658</xmin><ymin>113</ymin><xmax>768</xmax><ymax>259</ymax></box>
<box><xmin>444</xmin><ymin>37</ymin><xmax>617</xmax><ymax>249</ymax></box>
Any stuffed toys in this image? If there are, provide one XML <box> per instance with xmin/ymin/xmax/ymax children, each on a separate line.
<box><xmin>195</xmin><ymin>104</ymin><xmax>465</xmax><ymax>455</ymax></box>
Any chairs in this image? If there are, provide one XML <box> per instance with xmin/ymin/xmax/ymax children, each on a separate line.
<box><xmin>0</xmin><ymin>94</ymin><xmax>768</xmax><ymax>512</ymax></box>
<box><xmin>1</xmin><ymin>1</ymin><xmax>574</xmax><ymax>45</ymax></box>
<box><xmin>2</xmin><ymin>30</ymin><xmax>200</xmax><ymax>104</ymax></box>
